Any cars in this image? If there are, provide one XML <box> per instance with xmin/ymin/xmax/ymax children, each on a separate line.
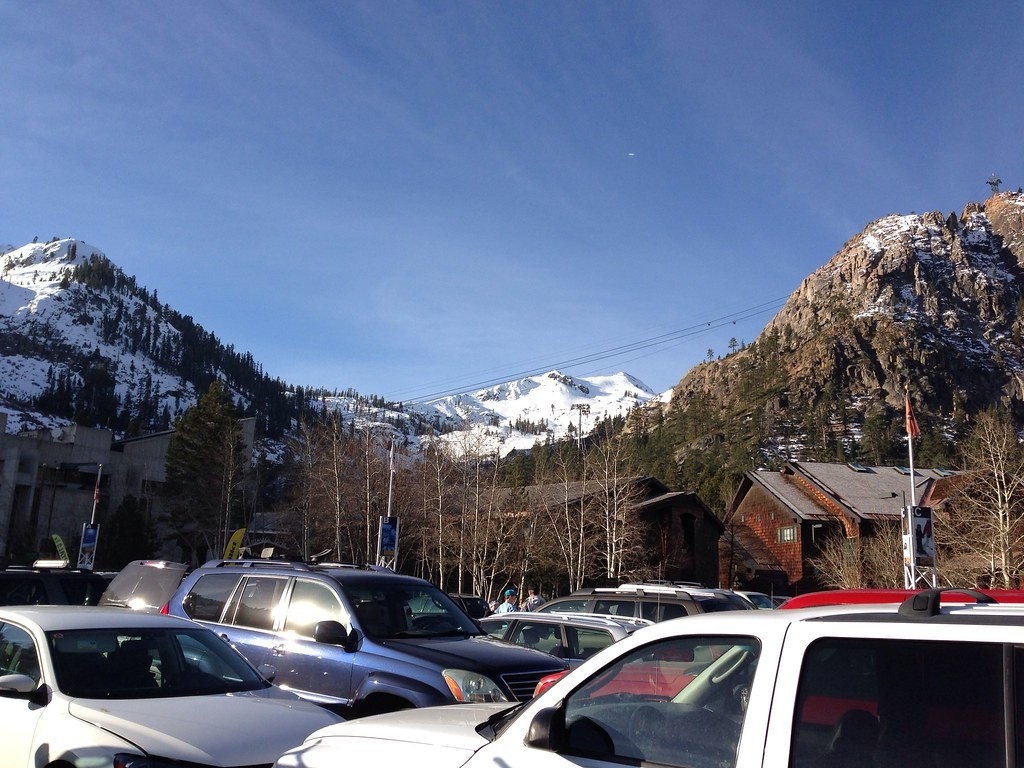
<box><xmin>0</xmin><ymin>602</ymin><xmax>345</xmax><ymax>768</ymax></box>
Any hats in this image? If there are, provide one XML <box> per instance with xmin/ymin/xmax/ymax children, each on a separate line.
<box><xmin>505</xmin><ymin>589</ymin><xmax>516</xmax><ymax>596</ymax></box>
<box><xmin>528</xmin><ymin>584</ymin><xmax>538</xmax><ymax>590</ymax></box>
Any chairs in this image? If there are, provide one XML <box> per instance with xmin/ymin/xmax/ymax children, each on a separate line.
<box><xmin>356</xmin><ymin>601</ymin><xmax>409</xmax><ymax>639</ymax></box>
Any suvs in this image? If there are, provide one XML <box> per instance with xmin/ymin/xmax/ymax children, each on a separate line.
<box><xmin>148</xmin><ymin>559</ymin><xmax>594</xmax><ymax>723</ymax></box>
<box><xmin>531</xmin><ymin>589</ymin><xmax>1023</xmax><ymax>710</ymax></box>
<box><xmin>405</xmin><ymin>579</ymin><xmax>794</xmax><ymax>717</ymax></box>
<box><xmin>270</xmin><ymin>584</ymin><xmax>1024</xmax><ymax>768</ymax></box>
<box><xmin>0</xmin><ymin>561</ymin><xmax>190</xmax><ymax>617</ymax></box>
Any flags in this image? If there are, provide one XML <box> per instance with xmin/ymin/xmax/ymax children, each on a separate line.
<box><xmin>93</xmin><ymin>481</ymin><xmax>100</xmax><ymax>503</ymax></box>
<box><xmin>906</xmin><ymin>394</ymin><xmax>921</xmax><ymax>439</ymax></box>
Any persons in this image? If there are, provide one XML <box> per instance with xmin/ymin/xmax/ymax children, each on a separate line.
<box><xmin>493</xmin><ymin>590</ymin><xmax>519</xmax><ymax>614</ymax></box>
<box><xmin>383</xmin><ymin>535</ymin><xmax>392</xmax><ymax>550</ymax></box>
<box><xmin>915</xmin><ymin>524</ymin><xmax>924</xmax><ymax>554</ymax></box>
<box><xmin>923</xmin><ymin>517</ymin><xmax>933</xmax><ymax>550</ymax></box>
<box><xmin>520</xmin><ymin>583</ymin><xmax>547</xmax><ymax>612</ymax></box>
<box><xmin>489</xmin><ymin>601</ymin><xmax>499</xmax><ymax>612</ymax></box>
